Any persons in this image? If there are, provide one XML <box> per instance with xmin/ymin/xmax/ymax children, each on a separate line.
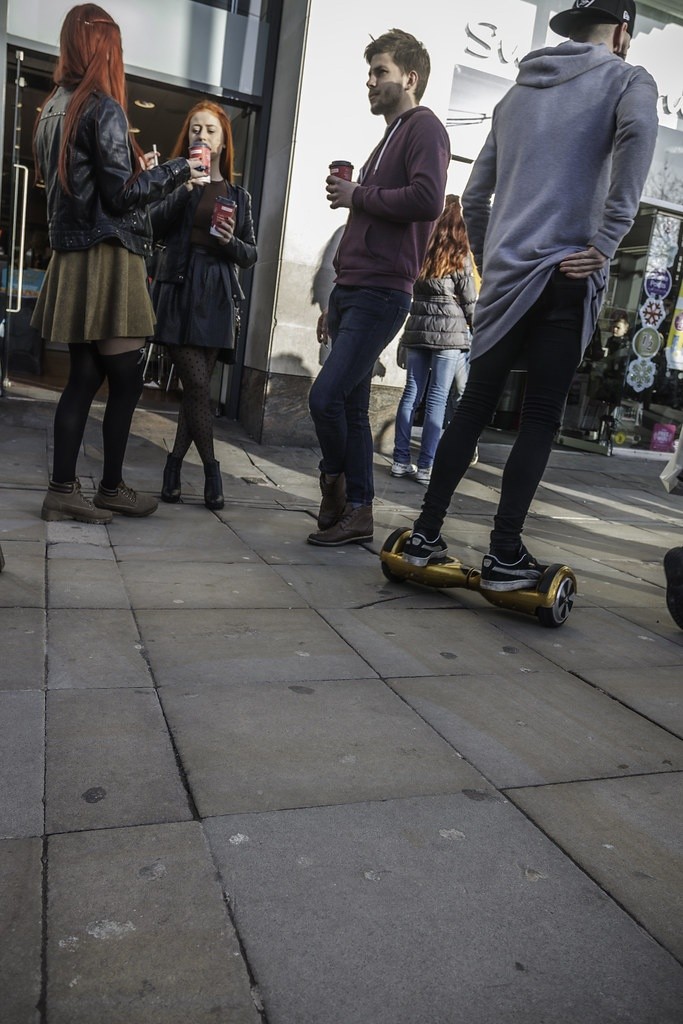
<box><xmin>29</xmin><ymin>3</ymin><xmax>257</xmax><ymax>523</ymax></box>
<box><xmin>391</xmin><ymin>193</ymin><xmax>479</xmax><ymax>483</ymax></box>
<box><xmin>577</xmin><ymin>319</ymin><xmax>631</xmax><ymax>432</ymax></box>
<box><xmin>308</xmin><ymin>28</ymin><xmax>450</xmax><ymax>546</ymax></box>
<box><xmin>405</xmin><ymin>0</ymin><xmax>658</xmax><ymax>592</ymax></box>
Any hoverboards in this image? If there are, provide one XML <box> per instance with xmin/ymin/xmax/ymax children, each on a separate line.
<box><xmin>379</xmin><ymin>527</ymin><xmax>580</xmax><ymax>629</ymax></box>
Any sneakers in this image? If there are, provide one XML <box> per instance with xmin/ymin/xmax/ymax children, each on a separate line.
<box><xmin>318</xmin><ymin>471</ymin><xmax>347</xmax><ymax>530</ymax></box>
<box><xmin>307</xmin><ymin>504</ymin><xmax>373</xmax><ymax>546</ymax></box>
<box><xmin>93</xmin><ymin>480</ymin><xmax>158</xmax><ymax>517</ymax></box>
<box><xmin>391</xmin><ymin>462</ymin><xmax>417</xmax><ymax>477</ymax></box>
<box><xmin>417</xmin><ymin>467</ymin><xmax>432</xmax><ymax>484</ymax></box>
<box><xmin>402</xmin><ymin>531</ymin><xmax>448</xmax><ymax>567</ymax></box>
<box><xmin>480</xmin><ymin>544</ymin><xmax>549</xmax><ymax>592</ymax></box>
<box><xmin>41</xmin><ymin>477</ymin><xmax>113</xmax><ymax>524</ymax></box>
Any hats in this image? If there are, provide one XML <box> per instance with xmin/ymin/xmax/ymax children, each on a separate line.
<box><xmin>549</xmin><ymin>0</ymin><xmax>636</xmax><ymax>38</ymax></box>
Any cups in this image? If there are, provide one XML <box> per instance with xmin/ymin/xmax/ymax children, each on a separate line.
<box><xmin>208</xmin><ymin>195</ymin><xmax>236</xmax><ymax>238</ymax></box>
<box><xmin>329</xmin><ymin>160</ymin><xmax>355</xmax><ymax>182</ymax></box>
<box><xmin>188</xmin><ymin>141</ymin><xmax>211</xmax><ymax>184</ymax></box>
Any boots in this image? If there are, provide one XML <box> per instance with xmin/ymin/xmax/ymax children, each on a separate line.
<box><xmin>162</xmin><ymin>453</ymin><xmax>182</xmax><ymax>503</ymax></box>
<box><xmin>204</xmin><ymin>460</ymin><xmax>225</xmax><ymax>510</ymax></box>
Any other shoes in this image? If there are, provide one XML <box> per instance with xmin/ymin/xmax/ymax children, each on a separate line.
<box><xmin>468</xmin><ymin>446</ymin><xmax>478</xmax><ymax>467</ymax></box>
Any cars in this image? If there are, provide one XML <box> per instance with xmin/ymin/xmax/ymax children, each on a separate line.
<box><xmin>567</xmin><ymin>345</ymin><xmax>683</xmax><ymax>407</ymax></box>
